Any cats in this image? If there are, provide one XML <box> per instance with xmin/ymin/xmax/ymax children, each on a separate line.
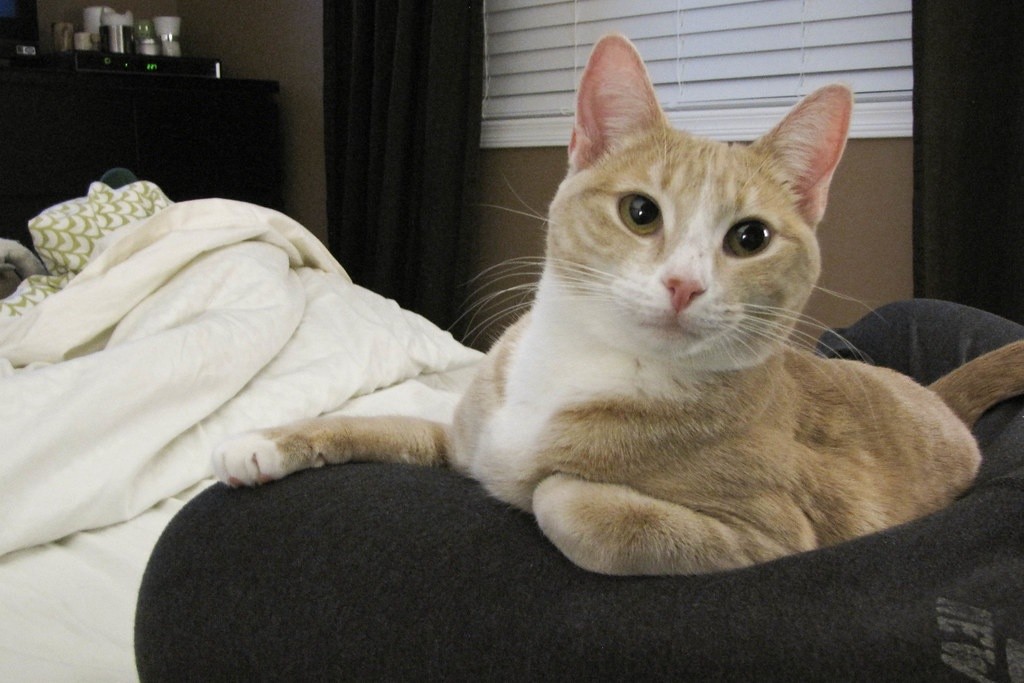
<box><xmin>205</xmin><ymin>32</ymin><xmax>1024</xmax><ymax>577</ymax></box>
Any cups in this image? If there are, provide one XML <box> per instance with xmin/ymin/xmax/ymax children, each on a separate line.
<box><xmin>72</xmin><ymin>33</ymin><xmax>93</xmax><ymax>52</ymax></box>
<box><xmin>51</xmin><ymin>20</ymin><xmax>74</xmax><ymax>53</ymax></box>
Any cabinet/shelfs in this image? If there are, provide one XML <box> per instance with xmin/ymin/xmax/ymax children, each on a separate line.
<box><xmin>0</xmin><ymin>64</ymin><xmax>284</xmax><ymax>256</ymax></box>
